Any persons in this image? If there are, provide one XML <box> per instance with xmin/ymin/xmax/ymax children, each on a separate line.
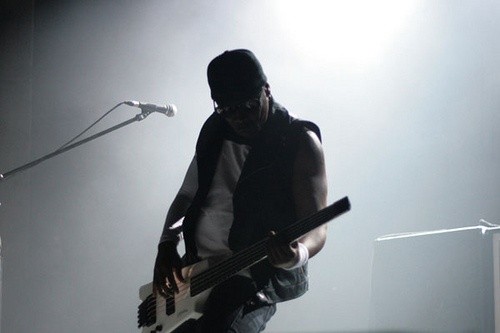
<box><xmin>153</xmin><ymin>49</ymin><xmax>327</xmax><ymax>333</ymax></box>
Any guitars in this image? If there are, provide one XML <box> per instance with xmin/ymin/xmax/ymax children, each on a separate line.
<box><xmin>137</xmin><ymin>196</ymin><xmax>351</xmax><ymax>333</ymax></box>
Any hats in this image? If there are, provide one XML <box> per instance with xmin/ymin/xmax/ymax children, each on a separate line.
<box><xmin>207</xmin><ymin>49</ymin><xmax>267</xmax><ymax>106</ymax></box>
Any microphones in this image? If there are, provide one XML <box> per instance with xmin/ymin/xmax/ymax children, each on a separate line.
<box><xmin>124</xmin><ymin>100</ymin><xmax>177</xmax><ymax>117</ymax></box>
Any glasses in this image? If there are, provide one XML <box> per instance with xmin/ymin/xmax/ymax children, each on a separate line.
<box><xmin>213</xmin><ymin>88</ymin><xmax>268</xmax><ymax>116</ymax></box>
